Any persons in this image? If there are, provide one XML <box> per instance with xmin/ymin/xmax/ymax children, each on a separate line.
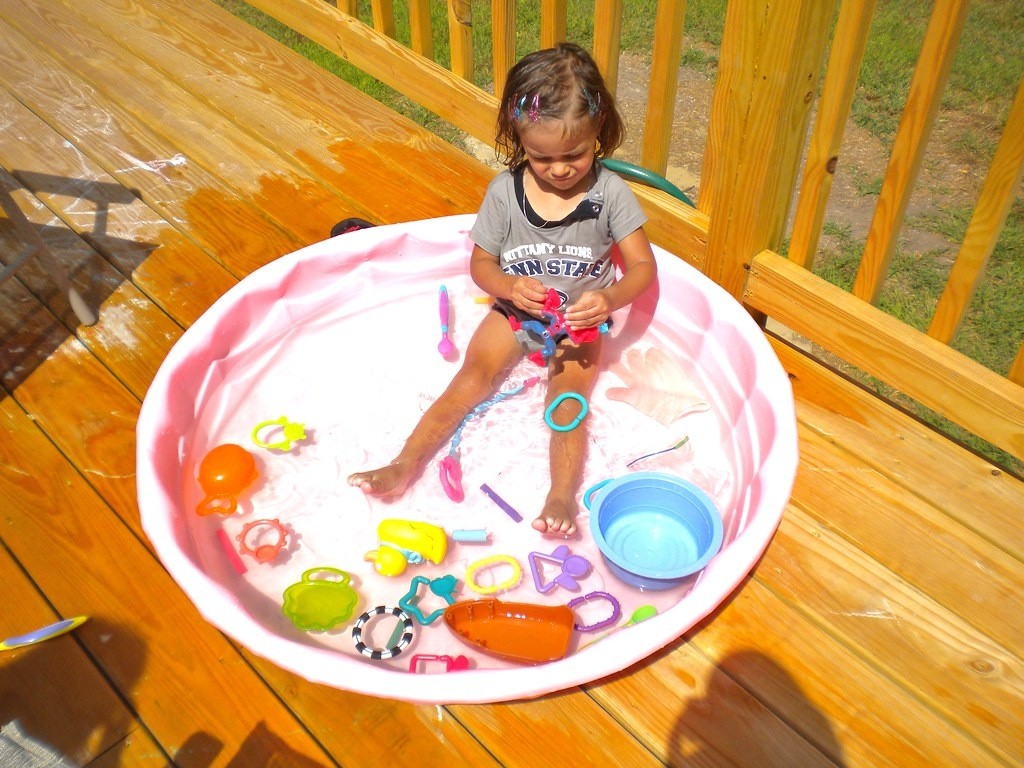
<box><xmin>348</xmin><ymin>40</ymin><xmax>657</xmax><ymax>538</ymax></box>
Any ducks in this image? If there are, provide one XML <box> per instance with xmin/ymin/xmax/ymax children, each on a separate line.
<box><xmin>365</xmin><ymin>519</ymin><xmax>448</xmax><ymax>578</ymax></box>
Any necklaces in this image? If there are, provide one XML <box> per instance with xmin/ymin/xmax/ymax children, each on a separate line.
<box><xmin>522</xmin><ymin>169</ymin><xmax>593</xmax><ymax>228</ymax></box>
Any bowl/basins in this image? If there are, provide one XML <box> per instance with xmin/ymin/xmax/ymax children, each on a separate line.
<box><xmin>584</xmin><ymin>471</ymin><xmax>725</xmax><ymax>590</ymax></box>
